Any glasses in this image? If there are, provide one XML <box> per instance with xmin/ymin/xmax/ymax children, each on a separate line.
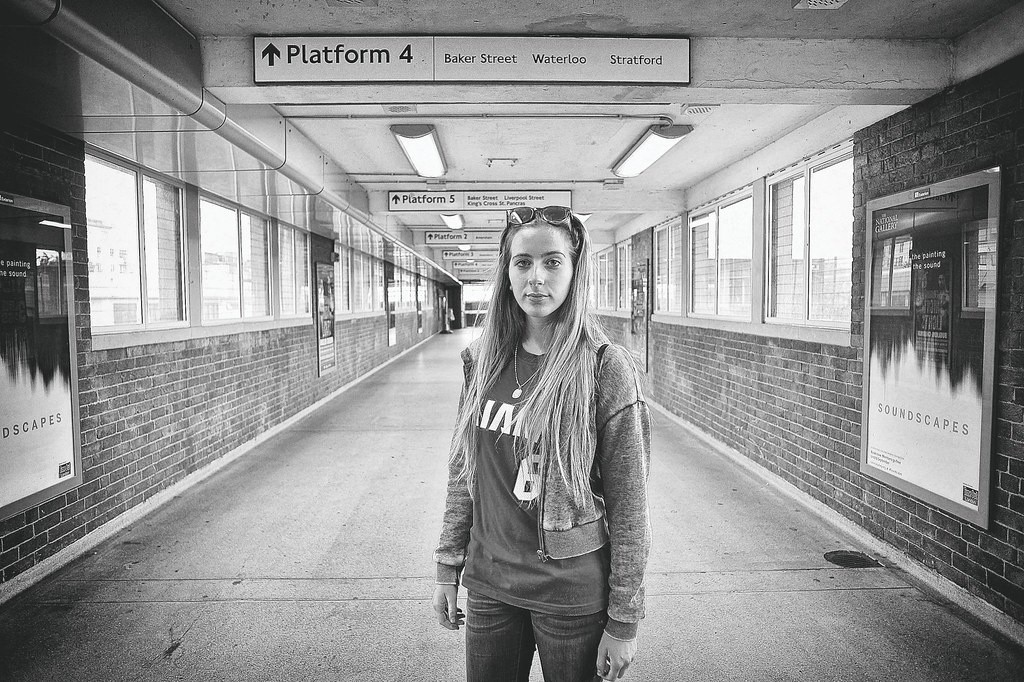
<box><xmin>507</xmin><ymin>206</ymin><xmax>576</xmax><ymax>247</ymax></box>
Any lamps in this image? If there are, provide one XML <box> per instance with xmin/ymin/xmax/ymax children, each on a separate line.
<box><xmin>611</xmin><ymin>124</ymin><xmax>694</xmax><ymax>179</ymax></box>
<box><xmin>439</xmin><ymin>214</ymin><xmax>465</xmax><ymax>229</ymax></box>
<box><xmin>389</xmin><ymin>123</ymin><xmax>449</xmax><ymax>178</ymax></box>
<box><xmin>574</xmin><ymin>213</ymin><xmax>592</xmax><ymax>224</ymax></box>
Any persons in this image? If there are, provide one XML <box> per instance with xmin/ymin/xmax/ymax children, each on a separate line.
<box><xmin>432</xmin><ymin>205</ymin><xmax>651</xmax><ymax>682</ymax></box>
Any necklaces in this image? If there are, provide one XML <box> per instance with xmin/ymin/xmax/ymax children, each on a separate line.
<box><xmin>512</xmin><ymin>337</ymin><xmax>542</xmax><ymax>399</ymax></box>
